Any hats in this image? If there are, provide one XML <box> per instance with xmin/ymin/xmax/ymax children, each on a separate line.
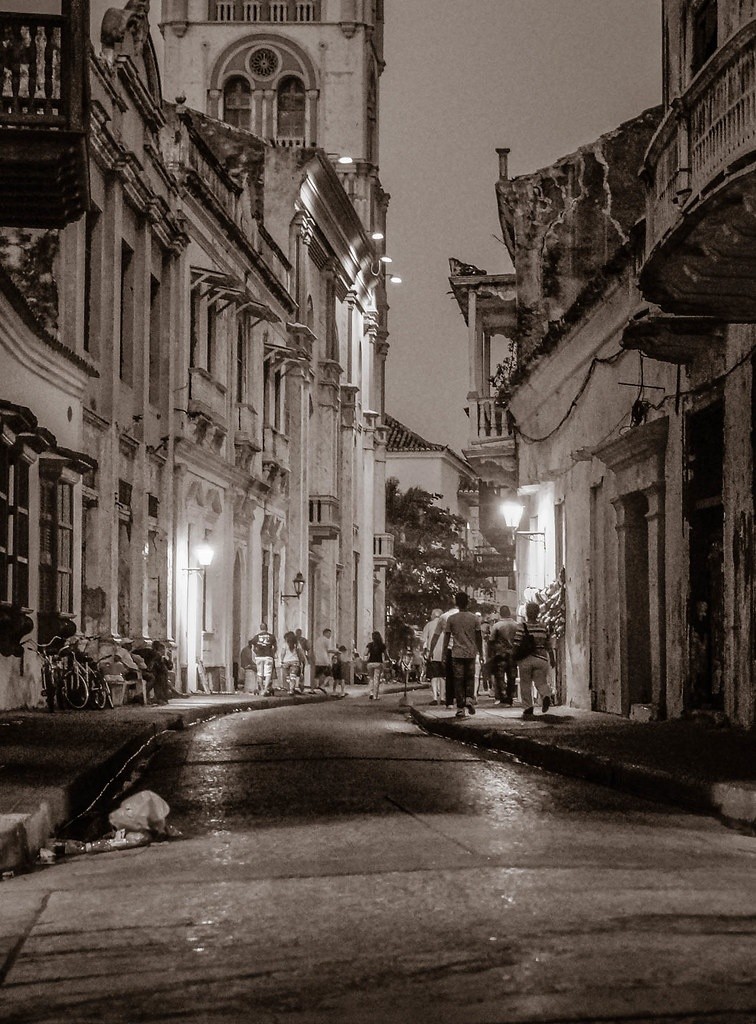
<box><xmin>120</xmin><ymin>638</ymin><xmax>134</xmax><ymax>645</ymax></box>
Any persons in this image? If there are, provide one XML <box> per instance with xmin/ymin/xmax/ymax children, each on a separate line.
<box><xmin>114</xmin><ymin>637</ymin><xmax>191</xmax><ymax>705</ymax></box>
<box><xmin>420</xmin><ymin>591</ymin><xmax>558</xmax><ymax>720</ymax></box>
<box><xmin>241</xmin><ymin>622</ymin><xmax>349</xmax><ymax>701</ymax></box>
<box><xmin>364</xmin><ymin>631</ymin><xmax>395</xmax><ymax>700</ymax></box>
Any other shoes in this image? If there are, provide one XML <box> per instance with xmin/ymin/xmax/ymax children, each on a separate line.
<box><xmin>466</xmin><ymin>697</ymin><xmax>475</xmax><ymax>714</ymax></box>
<box><xmin>375</xmin><ymin>696</ymin><xmax>379</xmax><ymax>700</ymax></box>
<box><xmin>318</xmin><ymin>687</ymin><xmax>327</xmax><ymax>693</ymax></box>
<box><xmin>369</xmin><ymin>694</ymin><xmax>373</xmax><ymax>699</ymax></box>
<box><xmin>524</xmin><ymin>706</ymin><xmax>533</xmax><ymax>714</ymax></box>
<box><xmin>309</xmin><ymin>691</ymin><xmax>316</xmax><ymax>694</ymax></box>
<box><xmin>456</xmin><ymin>708</ymin><xmax>465</xmax><ymax>717</ymax></box>
<box><xmin>543</xmin><ymin>696</ymin><xmax>550</xmax><ymax>712</ymax></box>
<box><xmin>446</xmin><ymin>705</ymin><xmax>453</xmax><ymax>709</ymax></box>
<box><xmin>440</xmin><ymin>701</ymin><xmax>446</xmax><ymax>704</ymax></box>
<box><xmin>430</xmin><ymin>700</ymin><xmax>437</xmax><ymax>705</ymax></box>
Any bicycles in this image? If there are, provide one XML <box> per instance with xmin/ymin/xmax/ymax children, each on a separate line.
<box><xmin>89</xmin><ymin>651</ymin><xmax>118</xmax><ymax>713</ymax></box>
<box><xmin>60</xmin><ymin>634</ymin><xmax>106</xmax><ymax>713</ymax></box>
<box><xmin>21</xmin><ymin>634</ymin><xmax>66</xmax><ymax>715</ymax></box>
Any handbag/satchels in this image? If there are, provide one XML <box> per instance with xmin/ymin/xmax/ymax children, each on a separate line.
<box><xmin>514</xmin><ymin>623</ymin><xmax>536</xmax><ymax>660</ymax></box>
<box><xmin>431</xmin><ymin>659</ymin><xmax>446</xmax><ymax>677</ymax></box>
<box><xmin>424</xmin><ymin>655</ymin><xmax>433</xmax><ymax>679</ymax></box>
<box><xmin>296</xmin><ymin>640</ymin><xmax>305</xmax><ymax>661</ymax></box>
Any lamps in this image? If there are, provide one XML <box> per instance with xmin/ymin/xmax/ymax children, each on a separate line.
<box><xmin>385</xmin><ymin>274</ymin><xmax>402</xmax><ymax>284</ymax></box>
<box><xmin>280</xmin><ymin>570</ymin><xmax>305</xmax><ymax>599</ymax></box>
<box><xmin>331</xmin><ymin>157</ymin><xmax>352</xmax><ymax>164</ymax></box>
<box><xmin>181</xmin><ymin>544</ymin><xmax>213</xmax><ymax>573</ymax></box>
<box><xmin>366</xmin><ymin>231</ymin><xmax>384</xmax><ymax>240</ymax></box>
<box><xmin>377</xmin><ymin>256</ymin><xmax>393</xmax><ymax>263</ymax></box>
<box><xmin>501</xmin><ymin>501</ymin><xmax>545</xmax><ymax>540</ymax></box>
